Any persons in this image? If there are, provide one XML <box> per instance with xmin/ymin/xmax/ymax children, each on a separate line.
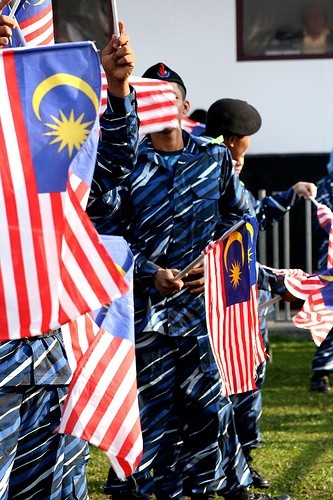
<box><xmin>311</xmin><ymin>172</ymin><xmax>333</xmax><ymax>393</ymax></box>
<box><xmin>0</xmin><ymin>0</ymin><xmax>318</xmax><ymax>500</ymax></box>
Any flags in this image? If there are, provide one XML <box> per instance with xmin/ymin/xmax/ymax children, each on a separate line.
<box><xmin>99</xmin><ymin>66</ymin><xmax>179</xmax><ymax>137</ymax></box>
<box><xmin>0</xmin><ymin>0</ymin><xmax>109</xmax><ymax>123</ymax></box>
<box><xmin>51</xmin><ymin>234</ymin><xmax>144</xmax><ymax>482</ymax></box>
<box><xmin>200</xmin><ymin>203</ymin><xmax>333</xmax><ymax>397</ymax></box>
<box><xmin>0</xmin><ymin>40</ymin><xmax>131</xmax><ymax>343</ymax></box>
<box><xmin>181</xmin><ymin>116</ymin><xmax>206</xmax><ymax>136</ymax></box>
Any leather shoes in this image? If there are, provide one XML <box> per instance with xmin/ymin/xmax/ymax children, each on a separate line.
<box><xmin>249</xmin><ymin>467</ymin><xmax>269</xmax><ymax>488</ymax></box>
<box><xmin>253</xmin><ymin>485</ymin><xmax>290</xmax><ymax>500</ymax></box>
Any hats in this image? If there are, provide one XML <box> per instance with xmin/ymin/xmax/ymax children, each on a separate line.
<box><xmin>142</xmin><ymin>63</ymin><xmax>187</xmax><ymax>99</ymax></box>
<box><xmin>205</xmin><ymin>98</ymin><xmax>261</xmax><ymax>136</ymax></box>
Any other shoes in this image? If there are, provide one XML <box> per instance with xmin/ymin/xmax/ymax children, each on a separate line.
<box><xmin>311</xmin><ymin>371</ymin><xmax>331</xmax><ymax>392</ymax></box>
